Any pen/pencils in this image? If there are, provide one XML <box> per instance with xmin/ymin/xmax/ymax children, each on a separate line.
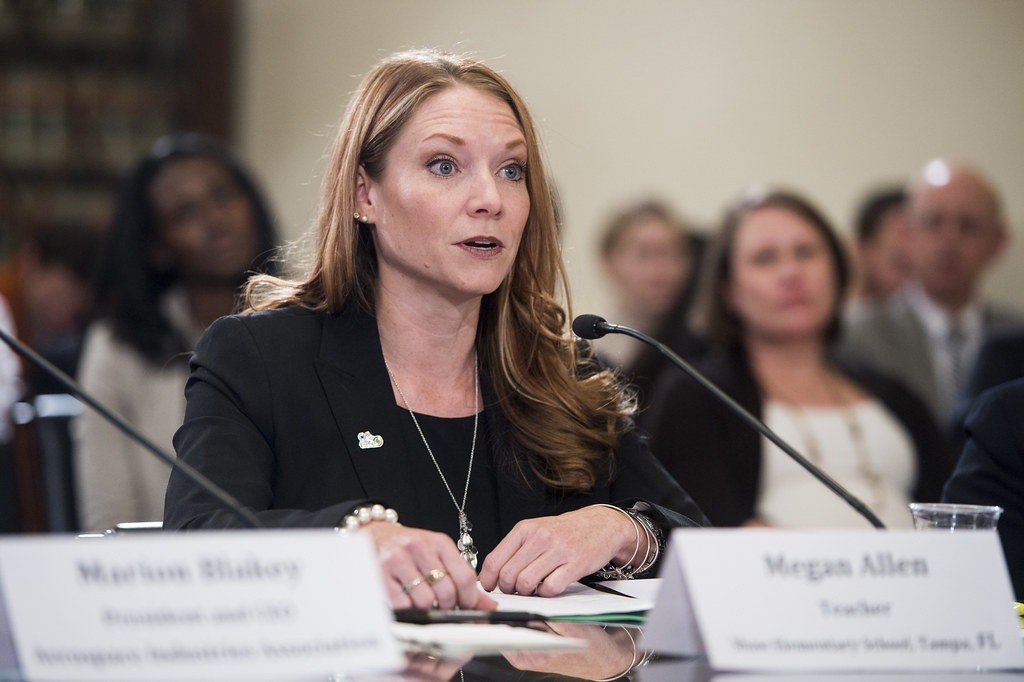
<box><xmin>394</xmin><ymin>606</ymin><xmax>553</xmax><ymax>624</ymax></box>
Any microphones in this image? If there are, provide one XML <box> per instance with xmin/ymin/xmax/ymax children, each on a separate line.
<box><xmin>570</xmin><ymin>315</ymin><xmax>882</xmax><ymax>528</ymax></box>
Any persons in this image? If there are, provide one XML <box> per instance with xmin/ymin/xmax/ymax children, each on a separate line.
<box><xmin>403</xmin><ymin>619</ymin><xmax>671</xmax><ymax>682</ymax></box>
<box><xmin>841</xmin><ymin>183</ymin><xmax>919</xmax><ymax>317</ymax></box>
<box><xmin>162</xmin><ymin>45</ymin><xmax>713</xmax><ymax>611</ymax></box>
<box><xmin>562</xmin><ymin>201</ymin><xmax>697</xmax><ymax>364</ymax></box>
<box><xmin>835</xmin><ymin>160</ymin><xmax>1022</xmax><ymax>431</ymax></box>
<box><xmin>624</xmin><ymin>179</ymin><xmax>957</xmax><ymax>525</ymax></box>
<box><xmin>74</xmin><ymin>134</ymin><xmax>286</xmax><ymax>539</ymax></box>
<box><xmin>937</xmin><ymin>376</ymin><xmax>1024</xmax><ymax>604</ymax></box>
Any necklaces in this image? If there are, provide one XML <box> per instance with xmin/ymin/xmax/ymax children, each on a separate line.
<box><xmin>381</xmin><ymin>342</ymin><xmax>482</xmax><ymax>575</ymax></box>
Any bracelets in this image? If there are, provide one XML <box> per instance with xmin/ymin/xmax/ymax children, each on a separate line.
<box><xmin>589</xmin><ymin>503</ymin><xmax>658</xmax><ymax>580</ymax></box>
<box><xmin>593</xmin><ymin>622</ymin><xmax>658</xmax><ymax>682</ymax></box>
<box><xmin>331</xmin><ymin>506</ymin><xmax>401</xmax><ymax>538</ymax></box>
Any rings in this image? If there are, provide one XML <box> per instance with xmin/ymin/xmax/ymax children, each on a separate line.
<box><xmin>420</xmin><ymin>654</ymin><xmax>438</xmax><ymax>665</ymax></box>
<box><xmin>422</xmin><ymin>565</ymin><xmax>447</xmax><ymax>588</ymax></box>
<box><xmin>403</xmin><ymin>571</ymin><xmax>425</xmax><ymax>595</ymax></box>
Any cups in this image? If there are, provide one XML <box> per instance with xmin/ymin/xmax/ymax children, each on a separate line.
<box><xmin>908</xmin><ymin>501</ymin><xmax>1004</xmax><ymax>532</ymax></box>
